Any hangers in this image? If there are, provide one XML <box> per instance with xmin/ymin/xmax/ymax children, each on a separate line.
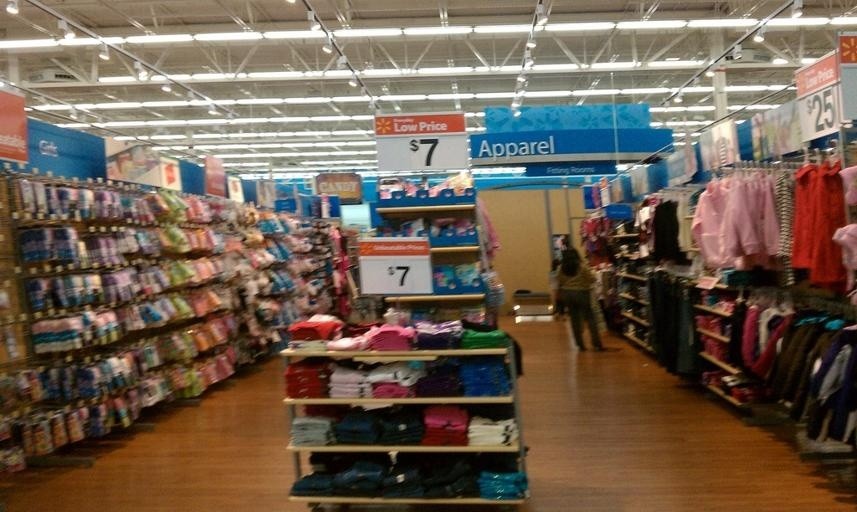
<box><xmin>750</xmin><ymin>286</ymin><xmax>857</xmax><ymax>331</ymax></box>
<box><xmin>658</xmin><ymin>187</ymin><xmax>693</xmax><ymax>202</ymax></box>
<box><xmin>659</xmin><ymin>264</ymin><xmax>698</xmax><ymax>280</ymax></box>
<box><xmin>712</xmin><ymin>160</ymin><xmax>806</xmax><ymax>178</ymax></box>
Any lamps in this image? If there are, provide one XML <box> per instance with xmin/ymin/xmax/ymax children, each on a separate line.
<box><xmin>0</xmin><ymin>0</ymin><xmax>857</xmax><ymax>171</ymax></box>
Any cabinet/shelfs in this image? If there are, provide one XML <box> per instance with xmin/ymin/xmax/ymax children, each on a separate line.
<box><xmin>374</xmin><ymin>203</ymin><xmax>490</xmax><ymax>301</ymax></box>
<box><xmin>690</xmin><ymin>273</ymin><xmax>756</xmax><ymax>417</ymax></box>
<box><xmin>608</xmin><ymin>230</ymin><xmax>651</xmax><ymax>358</ymax></box>
<box><xmin>280</xmin><ymin>344</ymin><xmax>530</xmax><ymax>511</ymax></box>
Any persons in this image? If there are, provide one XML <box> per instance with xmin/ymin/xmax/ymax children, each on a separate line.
<box><xmin>552</xmin><ymin>238</ymin><xmax>577</xmax><ymax>318</ymax></box>
<box><xmin>557</xmin><ymin>248</ymin><xmax>605</xmax><ymax>352</ymax></box>
